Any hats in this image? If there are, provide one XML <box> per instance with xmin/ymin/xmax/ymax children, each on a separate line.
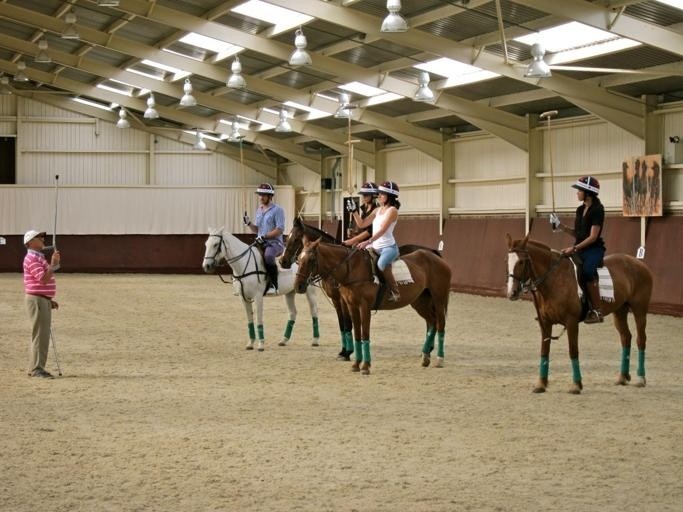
<box><xmin>23</xmin><ymin>230</ymin><xmax>47</xmax><ymax>245</ymax></box>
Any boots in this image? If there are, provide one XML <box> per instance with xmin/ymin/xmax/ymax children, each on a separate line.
<box><xmin>382</xmin><ymin>266</ymin><xmax>401</xmax><ymax>302</ymax></box>
<box><xmin>266</xmin><ymin>264</ymin><xmax>278</xmax><ymax>297</ymax></box>
<box><xmin>583</xmin><ymin>280</ymin><xmax>604</xmax><ymax>324</ymax></box>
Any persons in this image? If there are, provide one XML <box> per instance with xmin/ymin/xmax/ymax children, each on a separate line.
<box><xmin>347</xmin><ymin>181</ymin><xmax>402</xmax><ymax>302</ymax></box>
<box><xmin>243</xmin><ymin>183</ymin><xmax>285</xmax><ymax>295</ymax></box>
<box><xmin>344</xmin><ymin>184</ymin><xmax>379</xmax><ymax>247</ymax></box>
<box><xmin>24</xmin><ymin>231</ymin><xmax>61</xmax><ymax>379</ymax></box>
<box><xmin>550</xmin><ymin>175</ymin><xmax>606</xmax><ymax>316</ymax></box>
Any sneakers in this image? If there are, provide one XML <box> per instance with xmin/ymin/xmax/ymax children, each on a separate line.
<box><xmin>31</xmin><ymin>370</ymin><xmax>55</xmax><ymax>379</ymax></box>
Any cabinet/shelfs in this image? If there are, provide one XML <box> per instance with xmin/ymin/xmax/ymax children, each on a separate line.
<box><xmin>640</xmin><ymin>101</ymin><xmax>683</xmax><ymax>246</ymax></box>
<box><xmin>525</xmin><ymin>113</ymin><xmax>645</xmax><ymax>236</ymax></box>
<box><xmin>439</xmin><ymin>133</ymin><xmax>528</xmax><ymax>236</ymax></box>
<box><xmin>377</xmin><ymin>145</ymin><xmax>442</xmax><ymax>216</ymax></box>
<box><xmin>279</xmin><ymin>153</ymin><xmax>348</xmax><ymax>230</ymax></box>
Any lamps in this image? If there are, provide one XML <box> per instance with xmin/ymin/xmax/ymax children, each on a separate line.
<box><xmin>180</xmin><ymin>79</ymin><xmax>197</xmax><ymax>107</ymax></box>
<box><xmin>227</xmin><ymin>114</ymin><xmax>241</xmax><ymax>142</ymax></box>
<box><xmin>227</xmin><ymin>55</ymin><xmax>247</xmax><ymax>90</ymax></box>
<box><xmin>289</xmin><ymin>25</ymin><xmax>315</xmax><ymax>68</ymax></box>
<box><xmin>334</xmin><ymin>93</ymin><xmax>353</xmax><ymax>119</ymax></box>
<box><xmin>192</xmin><ymin>131</ymin><xmax>206</xmax><ymax>149</ymax></box>
<box><xmin>375</xmin><ymin>0</ymin><xmax>409</xmax><ymax>34</ymax></box>
<box><xmin>413</xmin><ymin>72</ymin><xmax>434</xmax><ymax>102</ymax></box>
<box><xmin>275</xmin><ymin>106</ymin><xmax>291</xmax><ymax>133</ymax></box>
<box><xmin>143</xmin><ymin>92</ymin><xmax>160</xmax><ymax>119</ymax></box>
<box><xmin>116</xmin><ymin>105</ymin><xmax>131</xmax><ymax>128</ymax></box>
<box><xmin>523</xmin><ymin>42</ymin><xmax>553</xmax><ymax>78</ymax></box>
<box><xmin>0</xmin><ymin>0</ymin><xmax>122</xmax><ymax>94</ymax></box>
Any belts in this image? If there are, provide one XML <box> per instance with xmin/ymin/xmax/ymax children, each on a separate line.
<box><xmin>27</xmin><ymin>293</ymin><xmax>52</xmax><ymax>300</ymax></box>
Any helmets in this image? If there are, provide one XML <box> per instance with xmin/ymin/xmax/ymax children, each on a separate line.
<box><xmin>377</xmin><ymin>180</ymin><xmax>399</xmax><ymax>198</ymax></box>
<box><xmin>356</xmin><ymin>182</ymin><xmax>379</xmax><ymax>195</ymax></box>
<box><xmin>254</xmin><ymin>183</ymin><xmax>274</xmax><ymax>195</ymax></box>
<box><xmin>571</xmin><ymin>176</ymin><xmax>600</xmax><ymax>196</ymax></box>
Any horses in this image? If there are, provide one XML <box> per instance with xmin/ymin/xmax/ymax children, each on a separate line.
<box><xmin>278</xmin><ymin>215</ymin><xmax>442</xmax><ymax>361</ymax></box>
<box><xmin>293</xmin><ymin>233</ymin><xmax>453</xmax><ymax>374</ymax></box>
<box><xmin>202</xmin><ymin>225</ymin><xmax>321</xmax><ymax>351</ymax></box>
<box><xmin>504</xmin><ymin>231</ymin><xmax>653</xmax><ymax>394</ymax></box>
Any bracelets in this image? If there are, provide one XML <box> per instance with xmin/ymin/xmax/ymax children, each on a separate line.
<box><xmin>366</xmin><ymin>240</ymin><xmax>370</xmax><ymax>243</ymax></box>
<box><xmin>573</xmin><ymin>246</ymin><xmax>577</xmax><ymax>251</ymax></box>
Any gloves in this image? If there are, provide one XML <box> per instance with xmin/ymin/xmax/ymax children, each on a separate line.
<box><xmin>255</xmin><ymin>235</ymin><xmax>265</xmax><ymax>245</ymax></box>
<box><xmin>550</xmin><ymin>212</ymin><xmax>561</xmax><ymax>229</ymax></box>
<box><xmin>242</xmin><ymin>211</ymin><xmax>251</xmax><ymax>226</ymax></box>
<box><xmin>346</xmin><ymin>196</ymin><xmax>357</xmax><ymax>212</ymax></box>
